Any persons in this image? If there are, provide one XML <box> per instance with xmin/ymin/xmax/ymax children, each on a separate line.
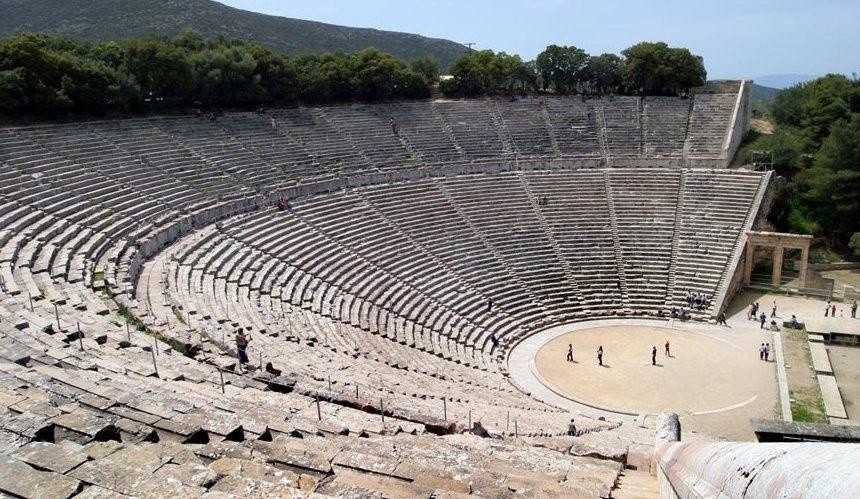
<box><xmin>474</xmin><ymin>421</ymin><xmax>492</xmax><ymax>438</ymax></box>
<box><xmin>850</xmin><ymin>299</ymin><xmax>858</xmax><ymax>318</ymax></box>
<box><xmin>236</xmin><ymin>326</ymin><xmax>253</xmax><ymax>369</ymax></box>
<box><xmin>831</xmin><ymin>304</ymin><xmax>837</xmax><ymax>318</ymax></box>
<box><xmin>597</xmin><ymin>345</ymin><xmax>604</xmax><ymax>366</ymax></box>
<box><xmin>490</xmin><ymin>333</ymin><xmax>500</xmax><ymax>347</ymax></box>
<box><xmin>759</xmin><ymin>342</ymin><xmax>765</xmax><ymax>360</ymax></box>
<box><xmin>747</xmin><ymin>298</ymin><xmax>798</xmax><ymax>330</ymax></box>
<box><xmin>664</xmin><ymin>340</ymin><xmax>670</xmax><ymax>357</ymax></box>
<box><xmin>657</xmin><ymin>289</ymin><xmax>710</xmax><ymax>321</ymax></box>
<box><xmin>651</xmin><ymin>346</ymin><xmax>657</xmax><ymax>365</ymax></box>
<box><xmin>487</xmin><ymin>297</ymin><xmax>493</xmax><ymax>312</ymax></box>
<box><xmin>567</xmin><ymin>343</ymin><xmax>574</xmax><ymax>361</ymax></box>
<box><xmin>541</xmin><ymin>196</ymin><xmax>549</xmax><ymax>205</ymax></box>
<box><xmin>536</xmin><ymin>195</ymin><xmax>542</xmax><ymax>205</ymax></box>
<box><xmin>719</xmin><ymin>310</ymin><xmax>727</xmax><ymax>327</ymax></box>
<box><xmin>839</xmin><ymin>310</ymin><xmax>846</xmax><ymax>319</ymax></box>
<box><xmin>251</xmin><ymin>194</ymin><xmax>293</xmax><ymax>213</ymax></box>
<box><xmin>566</xmin><ymin>418</ymin><xmax>577</xmax><ymax>436</ymax></box>
<box><xmin>764</xmin><ymin>343</ymin><xmax>770</xmax><ymax>362</ymax></box>
<box><xmin>824</xmin><ymin>300</ymin><xmax>831</xmax><ymax>317</ymax></box>
